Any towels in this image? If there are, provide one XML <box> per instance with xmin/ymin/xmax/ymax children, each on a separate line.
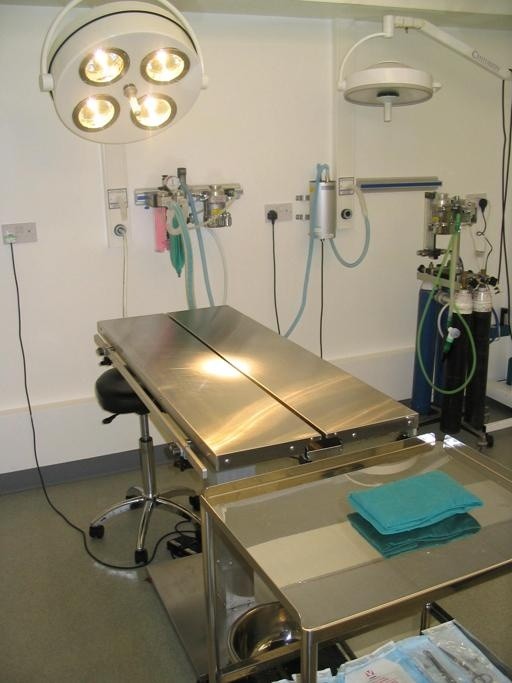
<box><xmin>349</xmin><ymin>470</ymin><xmax>483</xmax><ymax>536</ymax></box>
<box><xmin>346</xmin><ymin>512</ymin><xmax>481</xmax><ymax>560</ymax></box>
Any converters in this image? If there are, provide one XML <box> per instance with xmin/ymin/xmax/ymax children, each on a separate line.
<box><xmin>166</xmin><ymin>534</ymin><xmax>202</xmax><ymax>557</ymax></box>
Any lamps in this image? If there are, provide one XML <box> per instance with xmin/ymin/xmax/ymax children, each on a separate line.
<box><xmin>38</xmin><ymin>0</ymin><xmax>208</xmax><ymax>145</ymax></box>
<box><xmin>337</xmin><ymin>14</ymin><xmax>512</xmax><ymax>123</ymax></box>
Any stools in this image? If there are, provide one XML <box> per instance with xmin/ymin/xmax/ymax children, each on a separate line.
<box><xmin>88</xmin><ymin>366</ymin><xmax>201</xmax><ymax>563</ymax></box>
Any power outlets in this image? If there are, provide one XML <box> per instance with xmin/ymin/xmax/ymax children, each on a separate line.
<box><xmin>466</xmin><ymin>194</ymin><xmax>487</xmax><ymax>210</ymax></box>
<box><xmin>1</xmin><ymin>223</ymin><xmax>38</xmax><ymax>244</ymax></box>
<box><xmin>264</xmin><ymin>203</ymin><xmax>292</xmax><ymax>223</ymax></box>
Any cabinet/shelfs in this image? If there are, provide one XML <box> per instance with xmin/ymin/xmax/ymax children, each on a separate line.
<box><xmin>199</xmin><ymin>431</ymin><xmax>512</xmax><ymax>683</ymax></box>
